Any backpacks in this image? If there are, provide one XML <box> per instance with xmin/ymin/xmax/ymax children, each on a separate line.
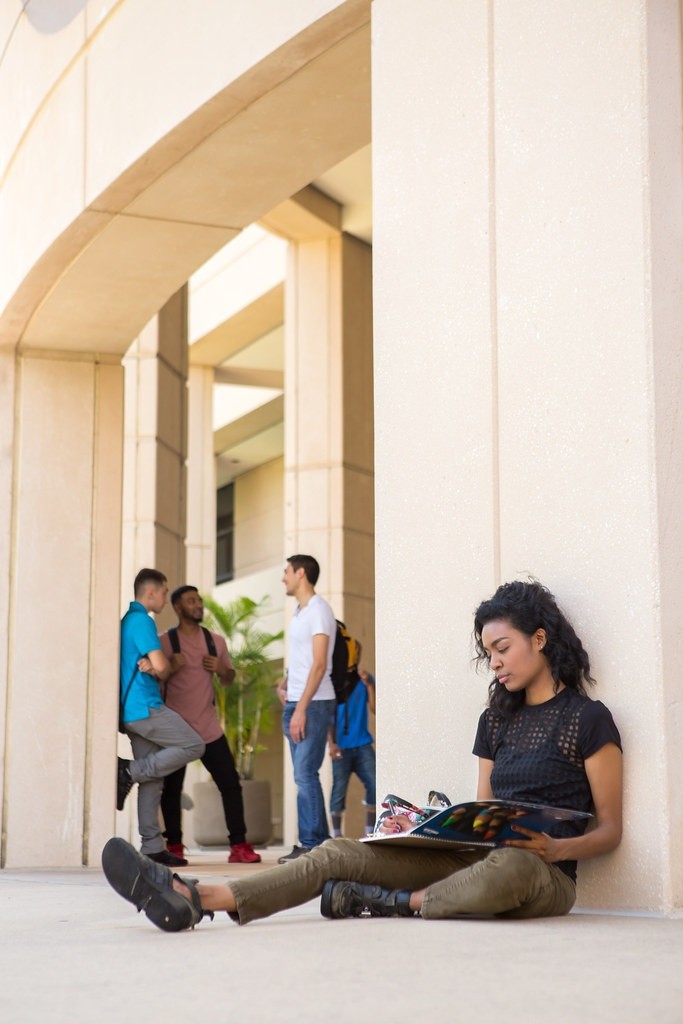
<box><xmin>332</xmin><ymin>620</ymin><xmax>362</xmax><ymax>703</ymax></box>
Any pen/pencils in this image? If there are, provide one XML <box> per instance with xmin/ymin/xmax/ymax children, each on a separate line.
<box><xmin>388</xmin><ymin>801</ymin><xmax>402</xmax><ymax>833</ymax></box>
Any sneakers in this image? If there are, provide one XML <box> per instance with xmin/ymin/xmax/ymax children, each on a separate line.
<box><xmin>278</xmin><ymin>846</ymin><xmax>310</xmax><ymax>864</ymax></box>
<box><xmin>145</xmin><ymin>851</ymin><xmax>188</xmax><ymax>866</ymax></box>
<box><xmin>228</xmin><ymin>842</ymin><xmax>260</xmax><ymax>863</ymax></box>
<box><xmin>166</xmin><ymin>843</ymin><xmax>184</xmax><ymax>858</ymax></box>
<box><xmin>117</xmin><ymin>757</ymin><xmax>134</xmax><ymax>811</ymax></box>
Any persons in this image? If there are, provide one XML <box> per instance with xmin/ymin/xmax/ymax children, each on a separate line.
<box><xmin>100</xmin><ymin>577</ymin><xmax>625</xmax><ymax>933</ymax></box>
<box><xmin>277</xmin><ymin>554</ymin><xmax>337</xmax><ymax>864</ymax></box>
<box><xmin>117</xmin><ymin>568</ymin><xmax>206</xmax><ymax>866</ymax></box>
<box><xmin>156</xmin><ymin>585</ymin><xmax>261</xmax><ymax>864</ymax></box>
<box><xmin>325</xmin><ymin>658</ymin><xmax>380</xmax><ymax>837</ymax></box>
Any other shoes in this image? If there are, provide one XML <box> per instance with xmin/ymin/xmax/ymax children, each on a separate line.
<box><xmin>321</xmin><ymin>879</ymin><xmax>413</xmax><ymax>918</ymax></box>
<box><xmin>102</xmin><ymin>838</ymin><xmax>202</xmax><ymax>932</ymax></box>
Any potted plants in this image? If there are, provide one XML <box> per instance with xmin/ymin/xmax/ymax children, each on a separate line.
<box><xmin>191</xmin><ymin>587</ymin><xmax>283</xmax><ymax>847</ymax></box>
